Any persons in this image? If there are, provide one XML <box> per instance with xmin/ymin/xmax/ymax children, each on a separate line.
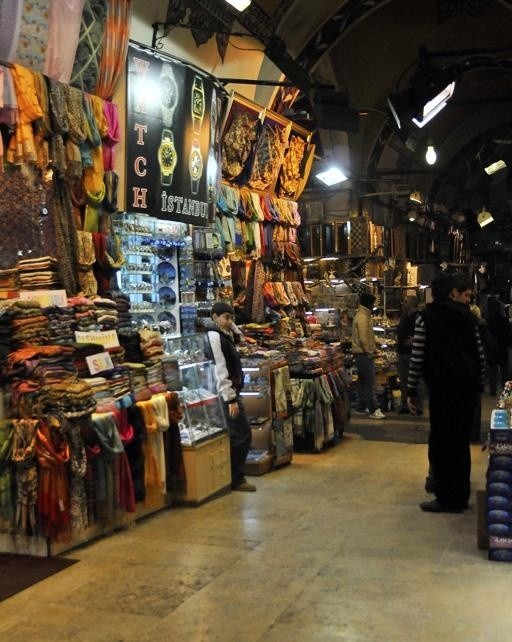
<box><xmin>485</xmin><ymin>298</ymin><xmax>512</xmax><ymax>395</ymax></box>
<box><xmin>396</xmin><ymin>294</ymin><xmax>425</xmax><ymax>417</ymax></box>
<box><xmin>407</xmin><ymin>271</ymin><xmax>485</xmax><ymax>514</ymax></box>
<box><xmin>449</xmin><ymin>271</ymin><xmax>476</xmax><ymax>304</ymax></box>
<box><xmin>352</xmin><ymin>293</ymin><xmax>386</xmax><ymax>419</ymax></box>
<box><xmin>204</xmin><ymin>302</ymin><xmax>258</xmax><ymax>492</ymax></box>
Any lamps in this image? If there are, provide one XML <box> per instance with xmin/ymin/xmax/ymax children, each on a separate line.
<box><xmin>406</xmin><ymin>69</ymin><xmax>458</xmax><ymax>129</ymax></box>
<box><xmin>477</xmin><ymin>206</ymin><xmax>493</xmax><ymax>228</ymax></box>
<box><xmin>315</xmin><ymin>167</ymin><xmax>349</xmax><ymax>187</ymax></box>
<box><xmin>409</xmin><ymin>191</ymin><xmax>425</xmax><ymax>204</ymax></box>
<box><xmin>484</xmin><ymin>160</ymin><xmax>506</xmax><ymax>177</ymax></box>
<box><xmin>425</xmin><ymin>138</ymin><xmax>437</xmax><ymax>166</ymax></box>
<box><xmin>225</xmin><ymin>0</ymin><xmax>252</xmax><ymax>12</ymax></box>
<box><xmin>385</xmin><ymin>89</ymin><xmax>413</xmax><ymax>133</ymax></box>
<box><xmin>314</xmin><ymin>103</ymin><xmax>360</xmax><ymax>133</ymax></box>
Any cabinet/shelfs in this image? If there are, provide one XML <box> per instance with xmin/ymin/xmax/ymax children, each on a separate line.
<box><xmin>115</xmin><ymin>219</ymin><xmax>158</xmax><ymax>331</ymax></box>
<box><xmin>161</xmin><ymin>332</ymin><xmax>232</xmax><ymax>507</ymax></box>
<box><xmin>240</xmin><ymin>357</ymin><xmax>291</xmax><ymax>476</ymax></box>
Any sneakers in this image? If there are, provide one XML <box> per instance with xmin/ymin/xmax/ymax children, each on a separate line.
<box><xmin>420</xmin><ymin>498</ymin><xmax>463</xmax><ymax>512</ymax></box>
<box><xmin>398</xmin><ymin>408</ymin><xmax>409</xmax><ymax>414</ymax></box>
<box><xmin>356</xmin><ymin>407</ymin><xmax>369</xmax><ymax>416</ymax></box>
<box><xmin>368</xmin><ymin>408</ymin><xmax>387</xmax><ymax>419</ymax></box>
<box><xmin>232</xmin><ymin>479</ymin><xmax>256</xmax><ymax>491</ymax></box>
